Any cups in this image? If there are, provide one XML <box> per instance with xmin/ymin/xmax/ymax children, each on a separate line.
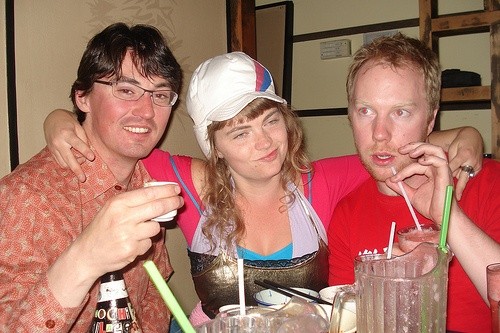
<box><xmin>396</xmin><ymin>222</ymin><xmax>442</xmax><ymax>251</ymax></box>
<box><xmin>486</xmin><ymin>263</ymin><xmax>500</xmax><ymax>333</ymax></box>
<box><xmin>215</xmin><ymin>307</ymin><xmax>279</xmax><ymax>323</ymax></box>
<box><xmin>353</xmin><ymin>254</ymin><xmax>396</xmax><ymax>265</ymax></box>
<box><xmin>147</xmin><ymin>181</ymin><xmax>178</xmax><ymax>222</ymax></box>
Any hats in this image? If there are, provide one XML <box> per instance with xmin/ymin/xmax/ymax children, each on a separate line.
<box><xmin>185</xmin><ymin>51</ymin><xmax>288</xmax><ymax>161</ymax></box>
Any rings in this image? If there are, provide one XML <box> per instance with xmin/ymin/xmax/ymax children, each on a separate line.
<box><xmin>462</xmin><ymin>166</ymin><xmax>474</xmax><ymax>177</ymax></box>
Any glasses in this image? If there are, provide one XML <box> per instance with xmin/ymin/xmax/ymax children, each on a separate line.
<box><xmin>94</xmin><ymin>79</ymin><xmax>178</xmax><ymax>108</ymax></box>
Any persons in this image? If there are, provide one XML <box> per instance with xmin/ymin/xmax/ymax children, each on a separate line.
<box><xmin>0</xmin><ymin>23</ymin><xmax>184</xmax><ymax>333</ymax></box>
<box><xmin>329</xmin><ymin>32</ymin><xmax>500</xmax><ymax>333</ymax></box>
<box><xmin>43</xmin><ymin>51</ymin><xmax>482</xmax><ymax>328</ymax></box>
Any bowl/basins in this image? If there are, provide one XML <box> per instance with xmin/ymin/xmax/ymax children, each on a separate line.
<box><xmin>319</xmin><ymin>285</ymin><xmax>356</xmax><ymax>312</ymax></box>
<box><xmin>252</xmin><ymin>288</ymin><xmax>319</xmax><ymax>308</ymax></box>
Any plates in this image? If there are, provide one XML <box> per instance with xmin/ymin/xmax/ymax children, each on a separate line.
<box><xmin>219</xmin><ymin>303</ymin><xmax>247</xmax><ymax>312</ymax></box>
<box><xmin>269</xmin><ymin>304</ymin><xmax>357</xmax><ymax>333</ymax></box>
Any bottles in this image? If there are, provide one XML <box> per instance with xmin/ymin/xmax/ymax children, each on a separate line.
<box><xmin>86</xmin><ymin>269</ymin><xmax>143</xmax><ymax>333</ymax></box>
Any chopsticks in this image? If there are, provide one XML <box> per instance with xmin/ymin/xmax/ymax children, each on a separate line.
<box><xmin>254</xmin><ymin>279</ymin><xmax>334</xmax><ymax>306</ymax></box>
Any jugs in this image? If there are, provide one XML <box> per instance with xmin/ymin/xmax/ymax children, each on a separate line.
<box><xmin>191</xmin><ymin>298</ymin><xmax>330</xmax><ymax>333</ymax></box>
<box><xmin>329</xmin><ymin>241</ymin><xmax>454</xmax><ymax>333</ymax></box>
